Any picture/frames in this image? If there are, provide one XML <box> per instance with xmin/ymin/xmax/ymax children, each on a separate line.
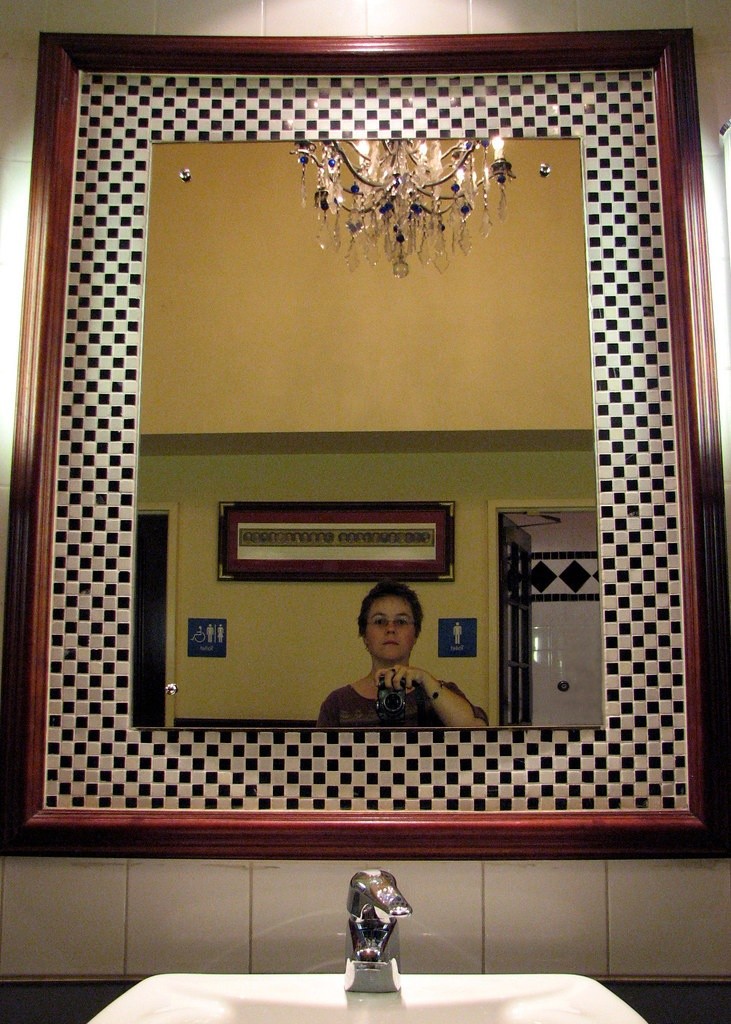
<box><xmin>218</xmin><ymin>502</ymin><xmax>455</xmax><ymax>582</ymax></box>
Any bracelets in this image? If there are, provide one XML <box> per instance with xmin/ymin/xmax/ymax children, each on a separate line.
<box><xmin>431</xmin><ymin>681</ymin><xmax>444</xmax><ymax>702</ymax></box>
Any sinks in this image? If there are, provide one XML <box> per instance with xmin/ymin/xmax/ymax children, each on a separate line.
<box><xmin>86</xmin><ymin>975</ymin><xmax>650</xmax><ymax>1024</ymax></box>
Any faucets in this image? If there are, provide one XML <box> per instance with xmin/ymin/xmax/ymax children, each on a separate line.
<box><xmin>341</xmin><ymin>870</ymin><xmax>410</xmax><ymax>993</ymax></box>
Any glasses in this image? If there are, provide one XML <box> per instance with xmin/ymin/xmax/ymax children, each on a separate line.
<box><xmin>366</xmin><ymin>615</ymin><xmax>415</xmax><ymax>629</ymax></box>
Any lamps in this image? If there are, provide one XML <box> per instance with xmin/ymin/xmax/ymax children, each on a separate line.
<box><xmin>288</xmin><ymin>137</ymin><xmax>516</xmax><ymax>279</ymax></box>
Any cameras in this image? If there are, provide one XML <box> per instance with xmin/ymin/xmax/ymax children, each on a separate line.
<box><xmin>376</xmin><ymin>676</ymin><xmax>406</xmax><ymax>727</ymax></box>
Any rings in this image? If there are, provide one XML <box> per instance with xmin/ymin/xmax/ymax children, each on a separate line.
<box><xmin>391</xmin><ymin>668</ymin><xmax>395</xmax><ymax>673</ymax></box>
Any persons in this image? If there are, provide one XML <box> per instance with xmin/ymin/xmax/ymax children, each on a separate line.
<box><xmin>315</xmin><ymin>579</ymin><xmax>490</xmax><ymax>726</ymax></box>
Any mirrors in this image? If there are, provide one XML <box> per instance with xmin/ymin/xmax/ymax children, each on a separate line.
<box><xmin>130</xmin><ymin>137</ymin><xmax>604</xmax><ymax>730</ymax></box>
